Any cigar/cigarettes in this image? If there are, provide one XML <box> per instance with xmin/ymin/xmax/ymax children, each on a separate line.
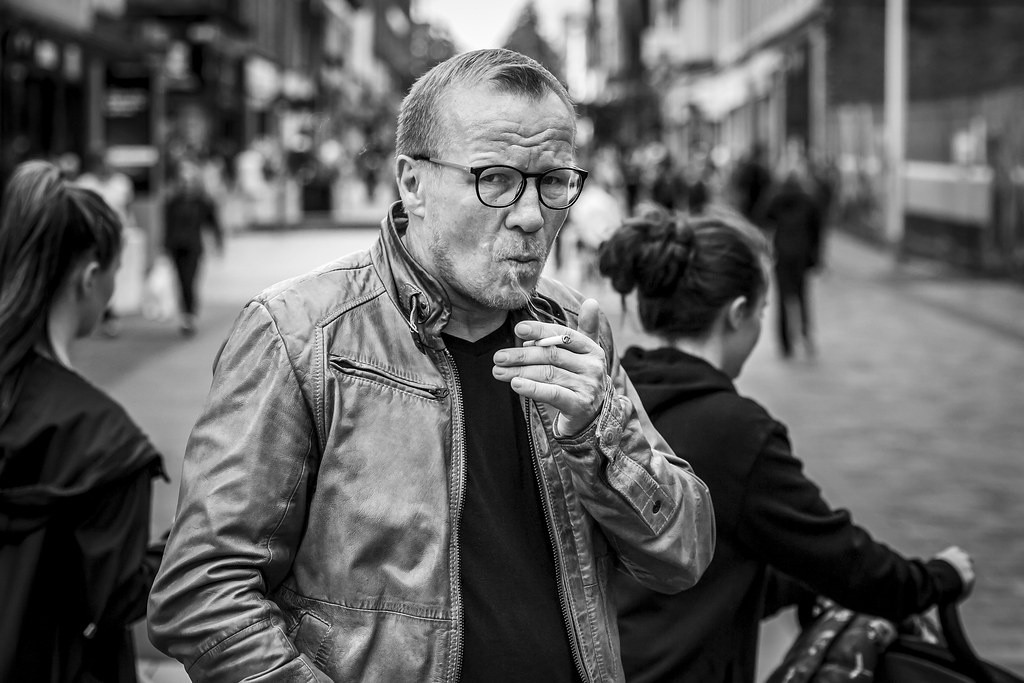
<box><xmin>523</xmin><ymin>334</ymin><xmax>573</xmax><ymax>347</ymax></box>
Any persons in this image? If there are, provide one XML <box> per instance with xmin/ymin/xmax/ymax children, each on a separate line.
<box><xmin>147</xmin><ymin>49</ymin><xmax>717</xmax><ymax>683</ymax></box>
<box><xmin>0</xmin><ymin>99</ymin><xmax>840</xmax><ymax>360</ymax></box>
<box><xmin>595</xmin><ymin>210</ymin><xmax>976</xmax><ymax>683</ymax></box>
<box><xmin>0</xmin><ymin>159</ymin><xmax>173</xmax><ymax>683</ymax></box>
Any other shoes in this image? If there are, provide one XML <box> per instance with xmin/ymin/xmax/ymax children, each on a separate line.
<box><xmin>180</xmin><ymin>322</ymin><xmax>198</xmax><ymax>334</ymax></box>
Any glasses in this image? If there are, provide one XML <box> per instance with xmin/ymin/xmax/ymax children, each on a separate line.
<box><xmin>411</xmin><ymin>155</ymin><xmax>588</xmax><ymax>210</ymax></box>
